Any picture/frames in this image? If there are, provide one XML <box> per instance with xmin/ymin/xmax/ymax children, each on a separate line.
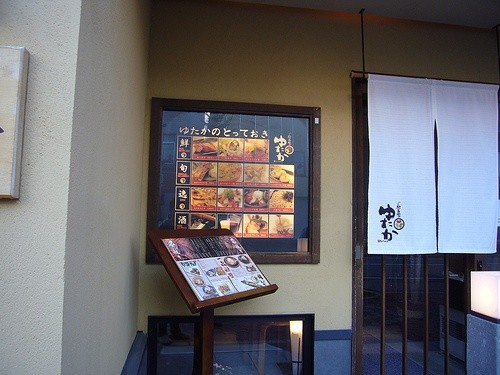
<box><xmin>145</xmin><ymin>96</ymin><xmax>320</xmax><ymax>266</ymax></box>
